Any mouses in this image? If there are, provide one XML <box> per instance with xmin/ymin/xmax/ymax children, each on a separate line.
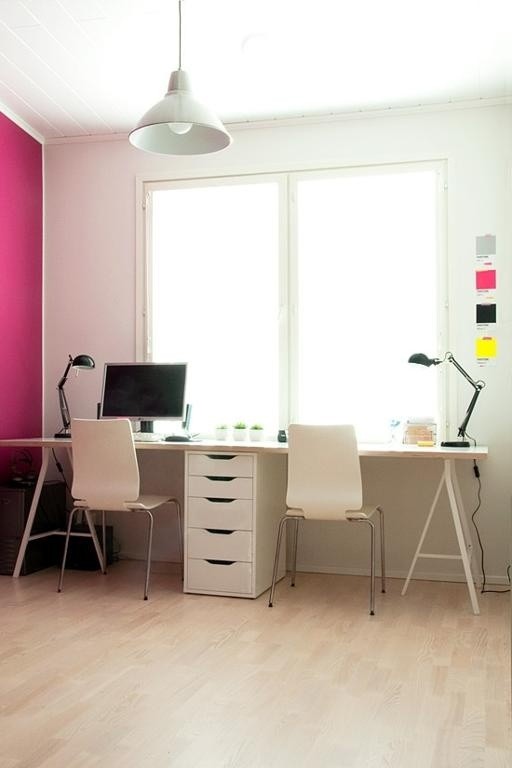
<box><xmin>165</xmin><ymin>435</ymin><xmax>190</xmax><ymax>441</ymax></box>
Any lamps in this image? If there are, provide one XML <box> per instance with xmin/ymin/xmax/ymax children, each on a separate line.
<box><xmin>128</xmin><ymin>1</ymin><xmax>234</xmax><ymax>159</ymax></box>
<box><xmin>54</xmin><ymin>353</ymin><xmax>95</xmax><ymax>439</ymax></box>
<box><xmin>407</xmin><ymin>350</ymin><xmax>486</xmax><ymax>448</ymax></box>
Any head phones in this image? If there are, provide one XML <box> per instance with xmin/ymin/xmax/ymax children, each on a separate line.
<box><xmin>10</xmin><ymin>449</ymin><xmax>36</xmax><ymax>486</ymax></box>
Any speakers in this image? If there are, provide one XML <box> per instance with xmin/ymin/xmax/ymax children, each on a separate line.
<box><xmin>65</xmin><ymin>524</ymin><xmax>113</xmax><ymax>572</ymax></box>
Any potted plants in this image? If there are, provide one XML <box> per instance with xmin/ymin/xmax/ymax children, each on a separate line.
<box><xmin>215</xmin><ymin>423</ymin><xmax>266</xmax><ymax>441</ymax></box>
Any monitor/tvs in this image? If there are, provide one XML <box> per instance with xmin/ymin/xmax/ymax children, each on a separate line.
<box><xmin>99</xmin><ymin>362</ymin><xmax>189</xmax><ymax>433</ymax></box>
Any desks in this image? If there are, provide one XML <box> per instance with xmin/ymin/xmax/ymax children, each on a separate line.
<box><xmin>1</xmin><ymin>431</ymin><xmax>488</xmax><ymax>615</ymax></box>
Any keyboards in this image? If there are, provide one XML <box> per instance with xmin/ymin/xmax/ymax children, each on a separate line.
<box><xmin>133</xmin><ymin>432</ymin><xmax>161</xmax><ymax>442</ymax></box>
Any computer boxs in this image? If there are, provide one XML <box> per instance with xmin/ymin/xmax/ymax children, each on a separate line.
<box><xmin>0</xmin><ymin>480</ymin><xmax>66</xmax><ymax>576</ymax></box>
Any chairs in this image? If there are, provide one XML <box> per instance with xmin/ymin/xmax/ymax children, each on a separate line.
<box><xmin>57</xmin><ymin>418</ymin><xmax>183</xmax><ymax>601</ymax></box>
<box><xmin>267</xmin><ymin>422</ymin><xmax>387</xmax><ymax>618</ymax></box>
